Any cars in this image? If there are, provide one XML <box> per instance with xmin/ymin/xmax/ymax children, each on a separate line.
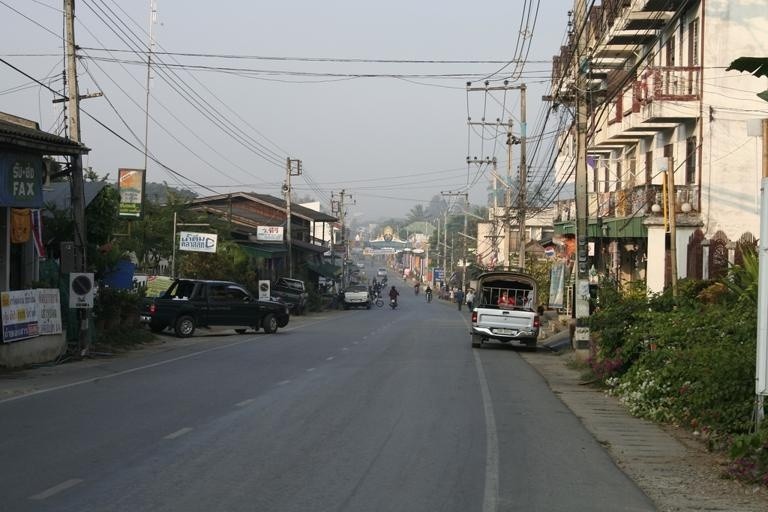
<box><xmin>355</xmin><ymin>260</ymin><xmax>364</xmax><ymax>268</ymax></box>
<box><xmin>377</xmin><ymin>267</ymin><xmax>388</xmax><ymax>276</ymax></box>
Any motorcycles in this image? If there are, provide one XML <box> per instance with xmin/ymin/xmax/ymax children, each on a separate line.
<box><xmin>425</xmin><ymin>290</ymin><xmax>432</xmax><ymax>301</ymax></box>
<box><xmin>415</xmin><ymin>287</ymin><xmax>419</xmax><ymax>295</ymax></box>
<box><xmin>371</xmin><ymin>279</ymin><xmax>398</xmax><ymax>310</ymax></box>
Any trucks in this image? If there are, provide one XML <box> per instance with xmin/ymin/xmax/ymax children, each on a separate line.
<box><xmin>468</xmin><ymin>270</ymin><xmax>543</xmax><ymax>351</ymax></box>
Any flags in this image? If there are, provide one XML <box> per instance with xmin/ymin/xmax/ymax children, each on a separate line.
<box><xmin>30</xmin><ymin>208</ymin><xmax>45</xmax><ymax>258</ymax></box>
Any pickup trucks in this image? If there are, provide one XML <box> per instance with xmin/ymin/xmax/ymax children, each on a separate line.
<box><xmin>138</xmin><ymin>278</ymin><xmax>290</xmax><ymax>338</ymax></box>
<box><xmin>270</xmin><ymin>277</ymin><xmax>310</xmax><ymax>316</ymax></box>
<box><xmin>343</xmin><ymin>285</ymin><xmax>371</xmax><ymax>310</ymax></box>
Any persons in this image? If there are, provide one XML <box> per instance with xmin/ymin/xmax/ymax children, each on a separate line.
<box><xmin>425</xmin><ymin>286</ymin><xmax>432</xmax><ymax>294</ymax></box>
<box><xmin>449</xmin><ymin>289</ymin><xmax>455</xmax><ymax>304</ymax></box>
<box><xmin>172</xmin><ymin>290</ymin><xmax>188</xmax><ymax>301</ymax></box>
<box><xmin>389</xmin><ymin>286</ymin><xmax>399</xmax><ymax>302</ymax></box>
<box><xmin>456</xmin><ymin>288</ymin><xmax>464</xmax><ymax>311</ymax></box>
<box><xmin>466</xmin><ymin>289</ymin><xmax>476</xmax><ymax>311</ymax></box>
<box><xmin>497</xmin><ymin>291</ymin><xmax>516</xmax><ymax>310</ymax></box>
